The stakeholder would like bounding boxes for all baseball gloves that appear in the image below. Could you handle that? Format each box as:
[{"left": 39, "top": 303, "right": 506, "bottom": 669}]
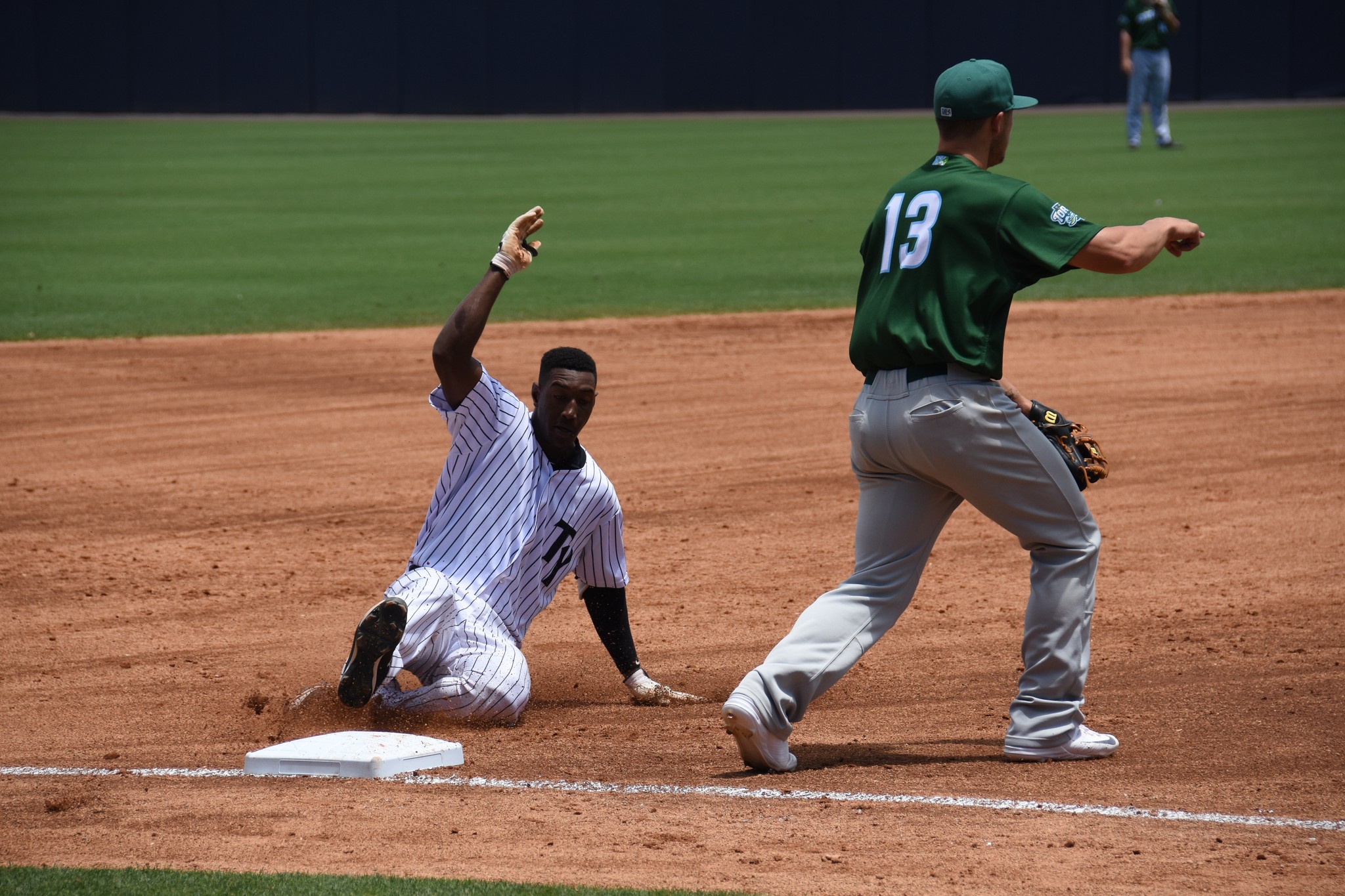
[{"left": 1031, "top": 399, "right": 1106, "bottom": 492}]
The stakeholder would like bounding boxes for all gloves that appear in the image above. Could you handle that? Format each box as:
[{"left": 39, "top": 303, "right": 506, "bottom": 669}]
[
  {"left": 490, "top": 206, "right": 544, "bottom": 280},
  {"left": 622, "top": 668, "right": 706, "bottom": 706}
]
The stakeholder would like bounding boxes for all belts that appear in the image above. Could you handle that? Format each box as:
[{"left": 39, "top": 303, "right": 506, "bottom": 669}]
[{"left": 863, "top": 362, "right": 947, "bottom": 386}]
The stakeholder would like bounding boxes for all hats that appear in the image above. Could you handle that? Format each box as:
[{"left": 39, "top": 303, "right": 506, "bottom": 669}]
[{"left": 933, "top": 59, "right": 1038, "bottom": 121}]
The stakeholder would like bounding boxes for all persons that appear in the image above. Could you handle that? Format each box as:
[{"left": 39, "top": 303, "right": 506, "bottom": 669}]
[
  {"left": 718, "top": 57, "right": 1205, "bottom": 774},
  {"left": 285, "top": 205, "right": 708, "bottom": 725},
  {"left": 1118, "top": 0, "right": 1185, "bottom": 150}
]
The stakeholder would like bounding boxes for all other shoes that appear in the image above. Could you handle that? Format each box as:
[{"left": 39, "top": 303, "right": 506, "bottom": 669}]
[
  {"left": 1129, "top": 143, "right": 1139, "bottom": 150},
  {"left": 1160, "top": 142, "right": 1182, "bottom": 149}
]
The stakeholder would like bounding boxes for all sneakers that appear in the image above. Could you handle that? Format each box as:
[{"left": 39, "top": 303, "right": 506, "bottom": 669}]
[
  {"left": 288, "top": 684, "right": 337, "bottom": 710},
  {"left": 722, "top": 693, "right": 797, "bottom": 773},
  {"left": 338, "top": 597, "right": 408, "bottom": 708},
  {"left": 1004, "top": 723, "right": 1119, "bottom": 762}
]
